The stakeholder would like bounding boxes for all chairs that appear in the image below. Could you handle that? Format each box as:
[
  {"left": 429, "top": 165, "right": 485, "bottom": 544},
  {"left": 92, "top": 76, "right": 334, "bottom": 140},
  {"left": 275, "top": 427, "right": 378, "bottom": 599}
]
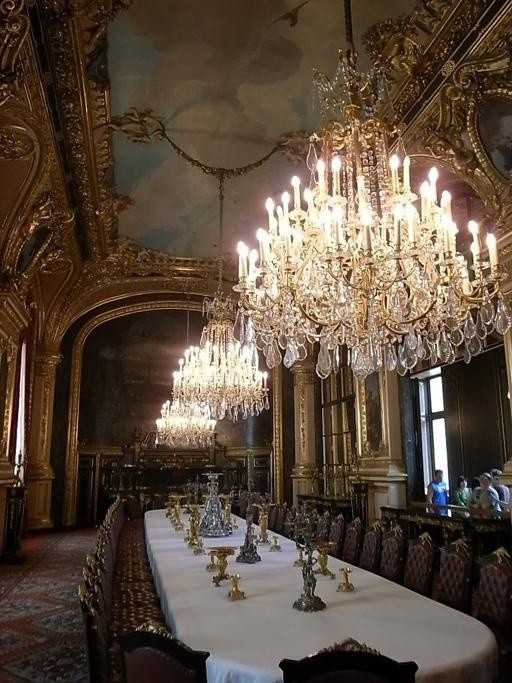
[
  {"left": 231, "top": 499, "right": 383, "bottom": 574},
  {"left": 463, "top": 546, "right": 512, "bottom": 667},
  {"left": 390, "top": 531, "right": 437, "bottom": 598},
  {"left": 116, "top": 619, "right": 210, "bottom": 683},
  {"left": 371, "top": 522, "right": 408, "bottom": 580},
  {"left": 75, "top": 492, "right": 172, "bottom": 683},
  {"left": 279, "top": 638, "right": 418, "bottom": 682},
  {"left": 417, "top": 534, "right": 475, "bottom": 613}
]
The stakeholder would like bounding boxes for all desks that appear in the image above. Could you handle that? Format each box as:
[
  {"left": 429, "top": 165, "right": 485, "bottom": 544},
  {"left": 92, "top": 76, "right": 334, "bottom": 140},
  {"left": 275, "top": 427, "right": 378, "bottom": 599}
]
[
  {"left": 381, "top": 504, "right": 511, "bottom": 586},
  {"left": 144, "top": 508, "right": 500, "bottom": 683}
]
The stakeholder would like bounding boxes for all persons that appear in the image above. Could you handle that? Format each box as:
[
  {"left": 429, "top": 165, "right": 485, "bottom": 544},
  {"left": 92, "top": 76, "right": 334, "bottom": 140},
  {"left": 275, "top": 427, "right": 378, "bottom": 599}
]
[
  {"left": 454, "top": 469, "right": 510, "bottom": 532},
  {"left": 427, "top": 470, "right": 448, "bottom": 517}
]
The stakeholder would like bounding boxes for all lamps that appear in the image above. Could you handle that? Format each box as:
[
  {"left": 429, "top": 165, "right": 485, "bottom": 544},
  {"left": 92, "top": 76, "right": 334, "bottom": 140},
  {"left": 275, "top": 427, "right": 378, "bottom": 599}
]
[{"left": 232, "top": 1, "right": 512, "bottom": 380}]
